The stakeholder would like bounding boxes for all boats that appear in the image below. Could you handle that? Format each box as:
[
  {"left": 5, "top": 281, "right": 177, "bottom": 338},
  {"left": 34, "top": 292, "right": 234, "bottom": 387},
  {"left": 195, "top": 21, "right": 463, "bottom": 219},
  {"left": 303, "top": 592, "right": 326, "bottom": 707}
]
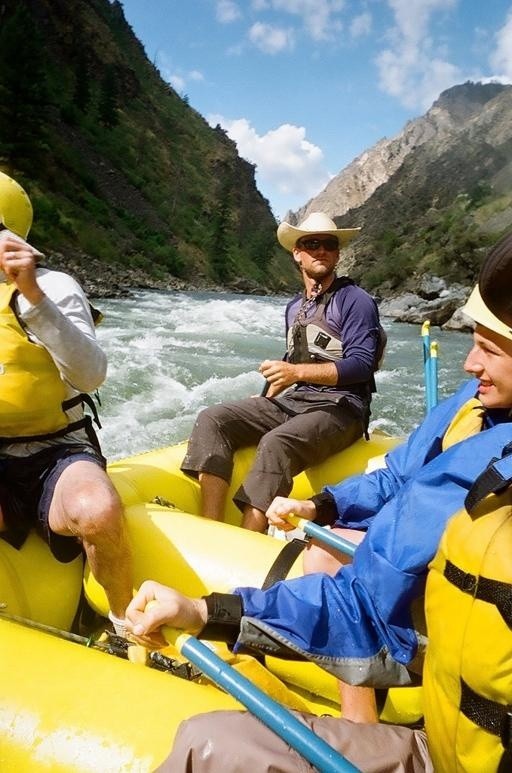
[{"left": 0, "top": 433, "right": 424, "bottom": 772}]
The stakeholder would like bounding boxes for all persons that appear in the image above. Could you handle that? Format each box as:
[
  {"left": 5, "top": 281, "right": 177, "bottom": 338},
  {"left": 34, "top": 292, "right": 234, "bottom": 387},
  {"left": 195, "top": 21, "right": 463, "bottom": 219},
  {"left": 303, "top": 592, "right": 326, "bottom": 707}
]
[
  {"left": 0, "top": 169, "right": 136, "bottom": 638},
  {"left": 123, "top": 420, "right": 512, "bottom": 773},
  {"left": 179, "top": 212, "right": 382, "bottom": 533},
  {"left": 263, "top": 220, "right": 511, "bottom": 725}
]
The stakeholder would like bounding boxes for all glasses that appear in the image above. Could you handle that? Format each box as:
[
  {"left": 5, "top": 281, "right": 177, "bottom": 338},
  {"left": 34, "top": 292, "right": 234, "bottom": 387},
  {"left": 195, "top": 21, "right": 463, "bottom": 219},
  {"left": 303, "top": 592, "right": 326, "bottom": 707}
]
[{"left": 297, "top": 238, "right": 339, "bottom": 251}]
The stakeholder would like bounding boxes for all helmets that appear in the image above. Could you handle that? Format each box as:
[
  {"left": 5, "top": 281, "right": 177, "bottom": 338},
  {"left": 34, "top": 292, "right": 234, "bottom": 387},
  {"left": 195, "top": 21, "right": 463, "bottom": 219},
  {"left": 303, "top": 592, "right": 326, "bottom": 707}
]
[
  {"left": 0, "top": 171, "right": 33, "bottom": 241},
  {"left": 461, "top": 226, "right": 512, "bottom": 339}
]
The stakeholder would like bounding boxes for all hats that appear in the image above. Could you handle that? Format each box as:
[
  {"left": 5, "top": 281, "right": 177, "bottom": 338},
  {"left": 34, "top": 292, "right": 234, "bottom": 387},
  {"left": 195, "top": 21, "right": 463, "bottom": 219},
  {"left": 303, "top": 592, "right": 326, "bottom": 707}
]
[{"left": 277, "top": 211, "right": 362, "bottom": 250}]
